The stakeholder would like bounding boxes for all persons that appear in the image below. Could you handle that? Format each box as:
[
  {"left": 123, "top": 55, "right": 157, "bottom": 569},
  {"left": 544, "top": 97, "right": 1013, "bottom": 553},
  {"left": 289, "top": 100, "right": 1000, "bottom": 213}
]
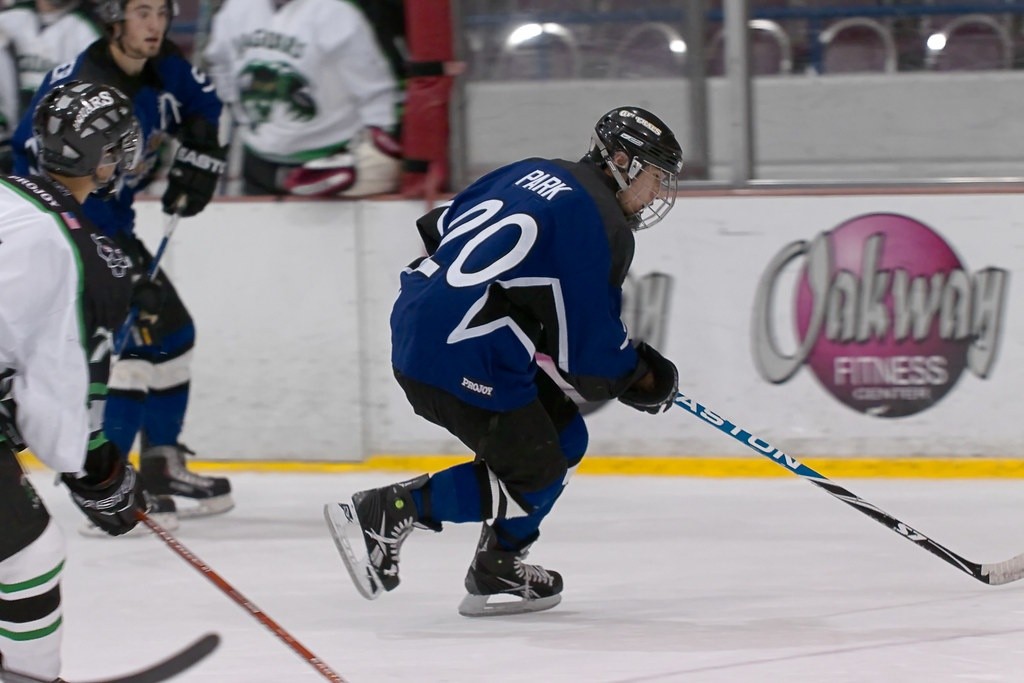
[
  {"left": 324, "top": 105, "right": 684, "bottom": 618},
  {"left": 0, "top": 81, "right": 145, "bottom": 683},
  {"left": 0, "top": 0, "right": 402, "bottom": 197},
  {"left": 12, "top": 0, "right": 234, "bottom": 538}
]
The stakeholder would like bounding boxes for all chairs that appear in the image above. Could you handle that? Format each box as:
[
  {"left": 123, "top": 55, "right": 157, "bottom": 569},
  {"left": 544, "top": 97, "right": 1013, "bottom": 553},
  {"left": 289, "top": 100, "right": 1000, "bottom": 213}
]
[{"left": 491, "top": 14, "right": 1014, "bottom": 79}]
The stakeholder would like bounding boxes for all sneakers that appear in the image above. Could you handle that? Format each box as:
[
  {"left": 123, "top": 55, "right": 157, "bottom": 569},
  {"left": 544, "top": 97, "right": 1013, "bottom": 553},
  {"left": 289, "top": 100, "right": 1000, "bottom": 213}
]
[
  {"left": 78, "top": 490, "right": 179, "bottom": 536},
  {"left": 324, "top": 473, "right": 443, "bottom": 600},
  {"left": 458, "top": 520, "right": 563, "bottom": 616},
  {"left": 139, "top": 443, "right": 233, "bottom": 516}
]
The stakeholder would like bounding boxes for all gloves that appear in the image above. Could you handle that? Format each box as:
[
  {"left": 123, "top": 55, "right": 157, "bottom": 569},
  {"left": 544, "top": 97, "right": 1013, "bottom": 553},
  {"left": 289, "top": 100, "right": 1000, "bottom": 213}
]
[
  {"left": 617, "top": 341, "right": 678, "bottom": 414},
  {"left": 62, "top": 455, "right": 147, "bottom": 536},
  {"left": 161, "top": 138, "right": 230, "bottom": 217}
]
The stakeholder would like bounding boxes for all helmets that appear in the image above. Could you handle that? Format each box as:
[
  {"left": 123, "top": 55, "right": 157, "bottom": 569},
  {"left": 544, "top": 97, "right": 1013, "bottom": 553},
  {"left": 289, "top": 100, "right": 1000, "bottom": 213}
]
[
  {"left": 34, "top": 79, "right": 140, "bottom": 177},
  {"left": 100, "top": 0, "right": 180, "bottom": 24},
  {"left": 591, "top": 106, "right": 683, "bottom": 232}
]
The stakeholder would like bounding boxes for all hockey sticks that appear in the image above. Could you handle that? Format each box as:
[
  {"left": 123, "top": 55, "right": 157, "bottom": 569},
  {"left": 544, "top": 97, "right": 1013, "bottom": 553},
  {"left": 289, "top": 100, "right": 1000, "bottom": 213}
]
[
  {"left": 674, "top": 392, "right": 1024, "bottom": 586},
  {"left": 136, "top": 514, "right": 349, "bottom": 683},
  {"left": 99, "top": 194, "right": 186, "bottom": 379}
]
